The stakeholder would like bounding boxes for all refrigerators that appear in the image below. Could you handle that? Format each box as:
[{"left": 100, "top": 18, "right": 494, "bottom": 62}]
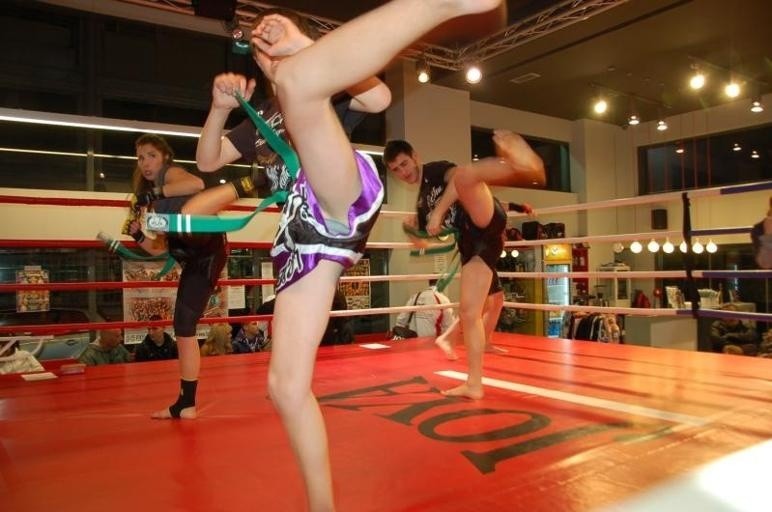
[{"left": 513, "top": 241, "right": 573, "bottom": 337}]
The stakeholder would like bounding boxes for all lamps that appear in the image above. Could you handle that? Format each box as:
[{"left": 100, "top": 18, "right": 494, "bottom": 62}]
[
  {"left": 747, "top": 91, "right": 767, "bottom": 114},
  {"left": 730, "top": 141, "right": 760, "bottom": 160},
  {"left": 610, "top": 108, "right": 722, "bottom": 256},
  {"left": 415, "top": 52, "right": 432, "bottom": 86}
]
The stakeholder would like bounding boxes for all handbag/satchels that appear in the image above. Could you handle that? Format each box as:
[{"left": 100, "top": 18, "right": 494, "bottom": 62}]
[{"left": 391, "top": 325, "right": 417, "bottom": 339}]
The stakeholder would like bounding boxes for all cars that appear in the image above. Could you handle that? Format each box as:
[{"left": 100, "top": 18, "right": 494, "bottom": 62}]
[{"left": 0, "top": 309, "right": 109, "bottom": 363}]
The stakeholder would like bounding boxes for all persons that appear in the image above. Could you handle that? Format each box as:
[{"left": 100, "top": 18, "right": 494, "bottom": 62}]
[
  {"left": 433, "top": 261, "right": 511, "bottom": 362},
  {"left": 195, "top": 1, "right": 502, "bottom": 511},
  {"left": 709, "top": 302, "right": 762, "bottom": 352},
  {"left": 393, "top": 279, "right": 461, "bottom": 337},
  {"left": 321, "top": 289, "right": 356, "bottom": 344},
  {"left": 134, "top": 315, "right": 178, "bottom": 362},
  {"left": 121, "top": 133, "right": 270, "bottom": 421},
  {"left": 750, "top": 196, "right": 772, "bottom": 270},
  {"left": 200, "top": 323, "right": 233, "bottom": 356},
  {"left": 383, "top": 128, "right": 548, "bottom": 402},
  {"left": 232, "top": 312, "right": 270, "bottom": 354},
  {"left": 77, "top": 328, "right": 136, "bottom": 367}
]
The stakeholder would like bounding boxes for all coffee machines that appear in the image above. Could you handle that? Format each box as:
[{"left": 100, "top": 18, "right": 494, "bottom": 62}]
[{"left": 593, "top": 285, "right": 606, "bottom": 306}]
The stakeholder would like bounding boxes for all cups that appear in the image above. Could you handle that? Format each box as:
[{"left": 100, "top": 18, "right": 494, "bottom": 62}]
[
  {"left": 684, "top": 302, "right": 692, "bottom": 309},
  {"left": 604, "top": 301, "right": 609, "bottom": 307}
]
